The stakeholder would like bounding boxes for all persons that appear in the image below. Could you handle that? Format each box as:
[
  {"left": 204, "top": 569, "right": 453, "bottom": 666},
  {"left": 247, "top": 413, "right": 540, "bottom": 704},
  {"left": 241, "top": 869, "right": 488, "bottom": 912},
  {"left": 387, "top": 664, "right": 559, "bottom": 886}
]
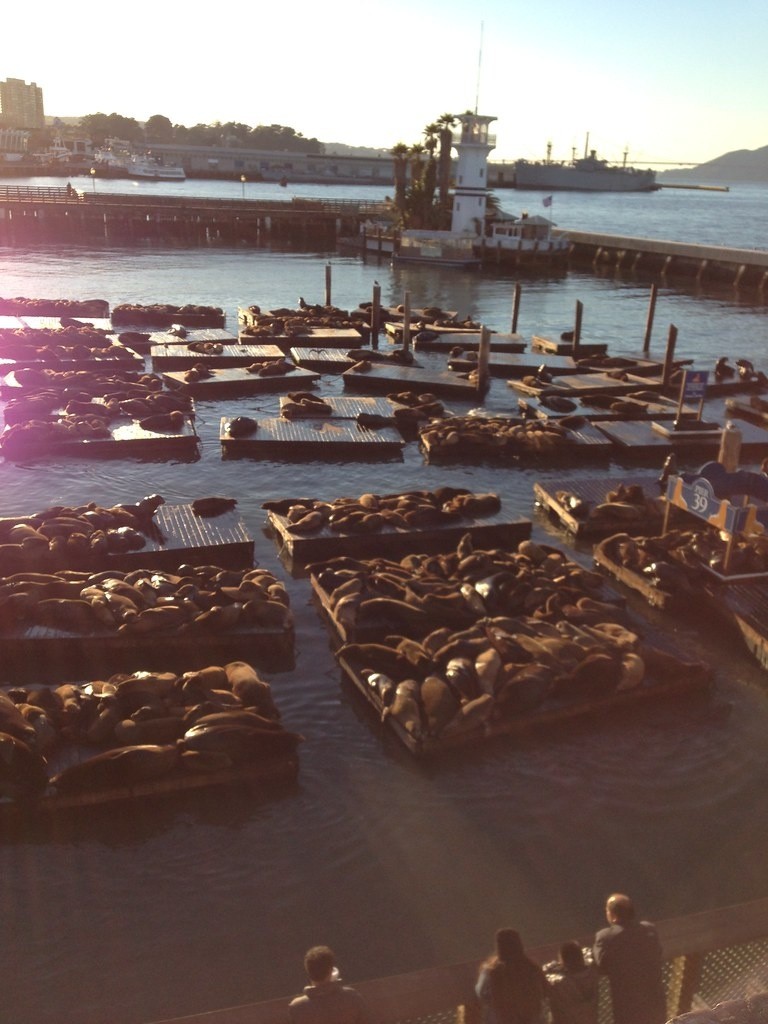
[
  {"left": 475, "top": 927, "right": 547, "bottom": 1024},
  {"left": 540, "top": 940, "right": 601, "bottom": 1024},
  {"left": 289, "top": 943, "right": 366, "bottom": 1024},
  {"left": 593, "top": 893, "right": 668, "bottom": 1024}
]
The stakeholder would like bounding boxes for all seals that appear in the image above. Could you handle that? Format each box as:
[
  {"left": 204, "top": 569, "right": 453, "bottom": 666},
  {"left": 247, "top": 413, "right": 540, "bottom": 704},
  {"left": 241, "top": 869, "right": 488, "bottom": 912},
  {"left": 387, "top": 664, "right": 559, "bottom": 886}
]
[{"left": 0, "top": 294, "right": 767, "bottom": 798}]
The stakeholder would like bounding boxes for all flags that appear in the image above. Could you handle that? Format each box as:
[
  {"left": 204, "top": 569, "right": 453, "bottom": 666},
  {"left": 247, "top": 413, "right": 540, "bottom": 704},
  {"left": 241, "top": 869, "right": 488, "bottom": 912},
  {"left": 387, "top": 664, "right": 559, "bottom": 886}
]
[{"left": 543, "top": 196, "right": 552, "bottom": 206}]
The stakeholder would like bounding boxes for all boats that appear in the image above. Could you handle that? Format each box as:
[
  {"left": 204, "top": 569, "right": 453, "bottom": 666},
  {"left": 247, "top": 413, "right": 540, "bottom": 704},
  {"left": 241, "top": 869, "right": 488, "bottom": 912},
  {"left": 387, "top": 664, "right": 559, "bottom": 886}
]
[
  {"left": 123, "top": 151, "right": 187, "bottom": 182},
  {"left": 515, "top": 132, "right": 656, "bottom": 193}
]
